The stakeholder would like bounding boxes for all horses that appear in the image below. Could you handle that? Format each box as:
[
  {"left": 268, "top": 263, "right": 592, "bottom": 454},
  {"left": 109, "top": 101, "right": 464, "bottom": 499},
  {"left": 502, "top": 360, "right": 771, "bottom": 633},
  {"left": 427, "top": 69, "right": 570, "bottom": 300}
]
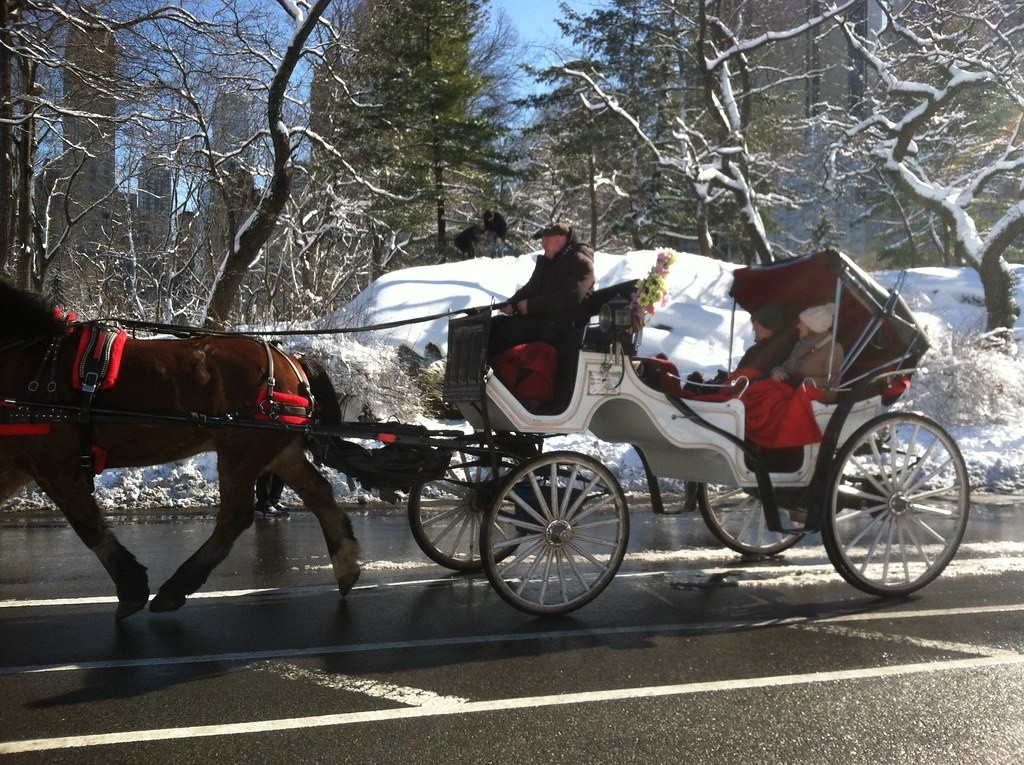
[{"left": 1, "top": 272, "right": 369, "bottom": 620}]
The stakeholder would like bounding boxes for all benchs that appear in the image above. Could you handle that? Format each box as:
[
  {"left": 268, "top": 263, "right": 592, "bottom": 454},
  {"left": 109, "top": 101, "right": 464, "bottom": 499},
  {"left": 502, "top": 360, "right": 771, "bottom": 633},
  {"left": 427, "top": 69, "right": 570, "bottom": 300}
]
[
  {"left": 554, "top": 279, "right": 642, "bottom": 392},
  {"left": 768, "top": 359, "right": 895, "bottom": 471}
]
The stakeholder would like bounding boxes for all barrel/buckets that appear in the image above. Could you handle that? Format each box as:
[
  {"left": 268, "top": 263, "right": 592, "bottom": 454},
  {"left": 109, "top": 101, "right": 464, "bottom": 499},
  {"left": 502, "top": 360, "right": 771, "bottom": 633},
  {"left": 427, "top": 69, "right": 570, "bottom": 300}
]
[{"left": 515, "top": 462, "right": 588, "bottom": 533}]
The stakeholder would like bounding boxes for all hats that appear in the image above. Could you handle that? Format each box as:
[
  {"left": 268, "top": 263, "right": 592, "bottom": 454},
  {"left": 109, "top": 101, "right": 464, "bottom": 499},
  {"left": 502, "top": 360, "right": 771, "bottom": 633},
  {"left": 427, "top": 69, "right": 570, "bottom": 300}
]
[
  {"left": 799, "top": 302, "right": 835, "bottom": 333},
  {"left": 532, "top": 222, "right": 570, "bottom": 239},
  {"left": 751, "top": 304, "right": 783, "bottom": 331}
]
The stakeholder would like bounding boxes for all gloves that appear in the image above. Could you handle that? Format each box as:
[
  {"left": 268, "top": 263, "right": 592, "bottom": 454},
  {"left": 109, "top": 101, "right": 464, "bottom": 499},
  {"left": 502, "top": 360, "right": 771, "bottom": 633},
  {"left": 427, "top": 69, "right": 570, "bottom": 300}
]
[{"left": 772, "top": 370, "right": 788, "bottom": 381}]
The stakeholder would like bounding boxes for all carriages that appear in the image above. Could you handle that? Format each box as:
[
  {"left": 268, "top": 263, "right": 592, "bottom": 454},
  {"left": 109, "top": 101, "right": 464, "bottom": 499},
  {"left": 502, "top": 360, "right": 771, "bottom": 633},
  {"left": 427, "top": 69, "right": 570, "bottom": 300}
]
[{"left": 0, "top": 242, "right": 974, "bottom": 624}]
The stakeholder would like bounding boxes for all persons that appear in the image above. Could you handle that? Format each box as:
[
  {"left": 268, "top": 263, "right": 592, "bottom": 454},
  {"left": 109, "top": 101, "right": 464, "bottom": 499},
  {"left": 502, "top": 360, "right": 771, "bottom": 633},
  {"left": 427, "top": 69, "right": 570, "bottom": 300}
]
[
  {"left": 771, "top": 303, "right": 845, "bottom": 402},
  {"left": 488, "top": 224, "right": 597, "bottom": 366},
  {"left": 454, "top": 224, "right": 484, "bottom": 259},
  {"left": 255, "top": 469, "right": 290, "bottom": 517},
  {"left": 483, "top": 209, "right": 507, "bottom": 258},
  {"left": 684, "top": 299, "right": 800, "bottom": 395}
]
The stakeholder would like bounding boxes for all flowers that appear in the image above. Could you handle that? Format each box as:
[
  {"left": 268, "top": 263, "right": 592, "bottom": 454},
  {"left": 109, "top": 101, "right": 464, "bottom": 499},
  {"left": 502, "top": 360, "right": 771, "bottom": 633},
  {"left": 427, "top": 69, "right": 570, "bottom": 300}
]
[{"left": 628, "top": 250, "right": 676, "bottom": 331}]
